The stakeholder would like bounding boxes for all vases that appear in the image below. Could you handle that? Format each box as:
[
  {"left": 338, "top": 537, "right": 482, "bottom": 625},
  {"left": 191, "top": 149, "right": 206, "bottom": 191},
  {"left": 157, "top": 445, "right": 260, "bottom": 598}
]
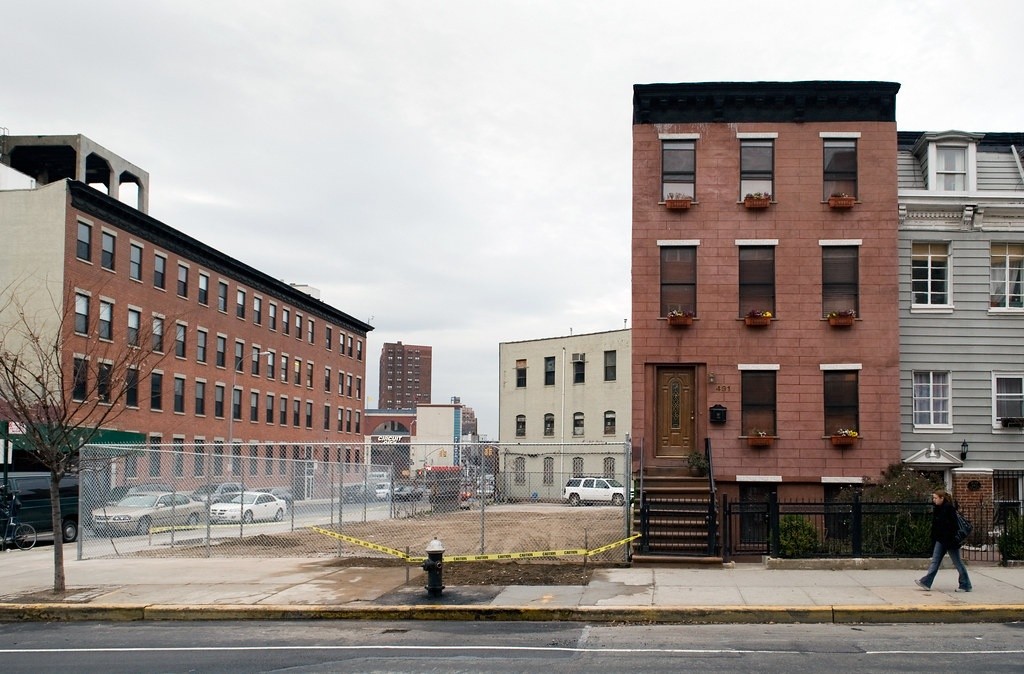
[
  {"left": 829, "top": 317, "right": 854, "bottom": 326},
  {"left": 667, "top": 317, "right": 693, "bottom": 325},
  {"left": 744, "top": 199, "right": 771, "bottom": 207},
  {"left": 827, "top": 197, "right": 855, "bottom": 207},
  {"left": 830, "top": 436, "right": 857, "bottom": 445},
  {"left": 744, "top": 317, "right": 771, "bottom": 325},
  {"left": 747, "top": 436, "right": 773, "bottom": 446}
]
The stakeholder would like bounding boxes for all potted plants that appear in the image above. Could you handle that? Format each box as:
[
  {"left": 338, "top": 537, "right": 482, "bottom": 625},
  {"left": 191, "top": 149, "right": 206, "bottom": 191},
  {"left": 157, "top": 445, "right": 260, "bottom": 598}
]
[
  {"left": 682, "top": 452, "right": 710, "bottom": 476},
  {"left": 666, "top": 193, "right": 693, "bottom": 209}
]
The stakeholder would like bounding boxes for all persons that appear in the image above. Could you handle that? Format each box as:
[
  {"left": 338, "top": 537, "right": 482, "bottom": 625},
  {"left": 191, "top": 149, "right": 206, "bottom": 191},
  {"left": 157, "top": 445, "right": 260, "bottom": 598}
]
[{"left": 915, "top": 489, "right": 973, "bottom": 593}]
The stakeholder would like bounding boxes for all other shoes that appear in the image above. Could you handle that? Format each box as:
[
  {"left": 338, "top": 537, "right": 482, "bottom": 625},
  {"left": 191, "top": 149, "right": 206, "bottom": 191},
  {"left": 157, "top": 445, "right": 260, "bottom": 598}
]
[
  {"left": 914, "top": 579, "right": 930, "bottom": 591},
  {"left": 955, "top": 588, "right": 972, "bottom": 592}
]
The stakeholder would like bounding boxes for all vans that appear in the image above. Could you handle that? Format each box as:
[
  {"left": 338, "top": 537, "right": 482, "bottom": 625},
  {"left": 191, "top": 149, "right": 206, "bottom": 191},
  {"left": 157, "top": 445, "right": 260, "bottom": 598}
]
[{"left": 0, "top": 471, "right": 79, "bottom": 543}]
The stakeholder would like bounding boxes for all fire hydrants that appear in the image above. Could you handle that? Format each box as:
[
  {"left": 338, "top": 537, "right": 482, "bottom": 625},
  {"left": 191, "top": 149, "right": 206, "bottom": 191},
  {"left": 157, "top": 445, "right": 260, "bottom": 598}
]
[{"left": 420, "top": 536, "right": 446, "bottom": 601}]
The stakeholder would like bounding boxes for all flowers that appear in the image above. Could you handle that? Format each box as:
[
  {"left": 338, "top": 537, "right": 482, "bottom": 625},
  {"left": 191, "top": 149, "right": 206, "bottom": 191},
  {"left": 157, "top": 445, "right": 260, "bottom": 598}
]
[
  {"left": 666, "top": 309, "right": 696, "bottom": 318},
  {"left": 832, "top": 428, "right": 858, "bottom": 437},
  {"left": 827, "top": 309, "right": 857, "bottom": 318},
  {"left": 830, "top": 192, "right": 849, "bottom": 198},
  {"left": 749, "top": 427, "right": 766, "bottom": 437},
  {"left": 746, "top": 308, "right": 772, "bottom": 316},
  {"left": 744, "top": 192, "right": 772, "bottom": 200}
]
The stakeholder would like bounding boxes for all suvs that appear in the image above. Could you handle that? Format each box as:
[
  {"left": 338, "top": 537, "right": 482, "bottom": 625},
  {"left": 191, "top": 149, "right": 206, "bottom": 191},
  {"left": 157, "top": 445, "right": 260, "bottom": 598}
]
[{"left": 562, "top": 475, "right": 634, "bottom": 507}]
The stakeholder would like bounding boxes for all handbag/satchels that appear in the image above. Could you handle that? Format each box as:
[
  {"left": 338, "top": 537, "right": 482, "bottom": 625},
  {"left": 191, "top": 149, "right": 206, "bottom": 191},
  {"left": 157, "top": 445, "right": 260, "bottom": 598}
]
[{"left": 954, "top": 513, "right": 973, "bottom": 544}]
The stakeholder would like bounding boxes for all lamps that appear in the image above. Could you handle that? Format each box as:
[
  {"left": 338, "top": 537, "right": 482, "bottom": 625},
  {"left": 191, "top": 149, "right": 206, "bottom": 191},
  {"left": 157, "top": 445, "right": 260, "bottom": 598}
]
[{"left": 959, "top": 439, "right": 968, "bottom": 461}]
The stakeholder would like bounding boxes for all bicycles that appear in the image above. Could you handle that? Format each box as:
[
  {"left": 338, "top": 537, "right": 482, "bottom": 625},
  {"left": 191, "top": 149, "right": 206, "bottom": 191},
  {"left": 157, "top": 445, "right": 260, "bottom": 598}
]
[{"left": 0, "top": 484, "right": 38, "bottom": 551}]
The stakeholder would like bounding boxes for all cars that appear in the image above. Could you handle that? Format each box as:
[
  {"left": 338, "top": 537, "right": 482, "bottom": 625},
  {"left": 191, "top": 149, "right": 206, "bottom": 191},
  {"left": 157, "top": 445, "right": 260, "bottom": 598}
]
[
  {"left": 190, "top": 481, "right": 293, "bottom": 510},
  {"left": 339, "top": 481, "right": 424, "bottom": 504},
  {"left": 208, "top": 491, "right": 287, "bottom": 524},
  {"left": 461, "top": 474, "right": 494, "bottom": 501},
  {"left": 91, "top": 490, "right": 206, "bottom": 538}
]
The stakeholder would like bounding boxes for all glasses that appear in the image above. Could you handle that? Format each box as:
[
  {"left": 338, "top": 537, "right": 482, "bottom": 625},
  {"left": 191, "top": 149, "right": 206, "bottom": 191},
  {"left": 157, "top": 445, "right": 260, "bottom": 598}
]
[{"left": 933, "top": 496, "right": 940, "bottom": 499}]
